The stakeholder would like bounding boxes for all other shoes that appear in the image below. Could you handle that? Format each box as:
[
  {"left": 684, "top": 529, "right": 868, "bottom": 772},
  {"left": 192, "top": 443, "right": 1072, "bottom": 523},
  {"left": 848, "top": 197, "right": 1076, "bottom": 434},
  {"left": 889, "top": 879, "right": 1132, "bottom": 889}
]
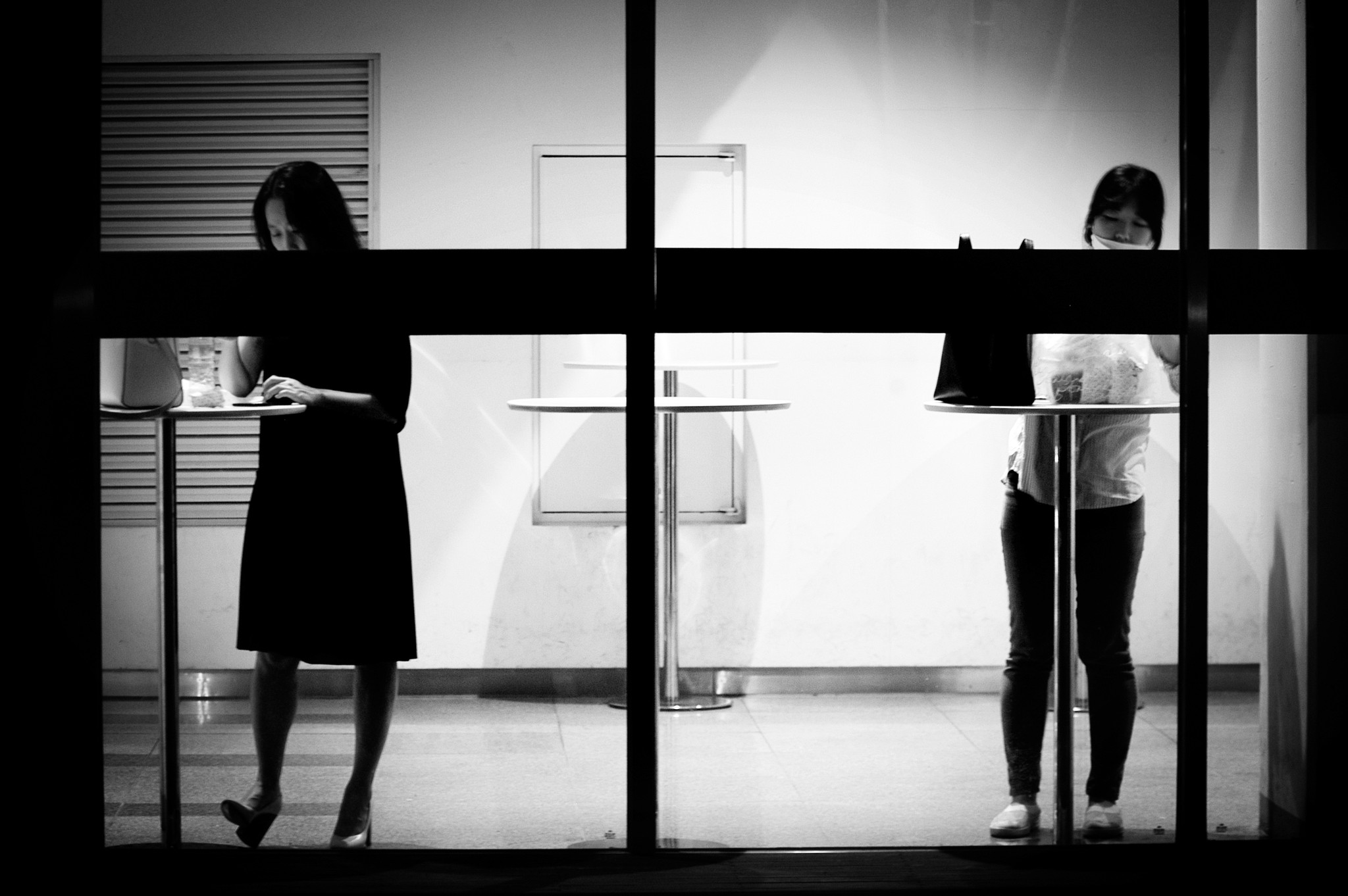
[
  {"left": 1083, "top": 800, "right": 1124, "bottom": 838},
  {"left": 989, "top": 800, "right": 1041, "bottom": 838}
]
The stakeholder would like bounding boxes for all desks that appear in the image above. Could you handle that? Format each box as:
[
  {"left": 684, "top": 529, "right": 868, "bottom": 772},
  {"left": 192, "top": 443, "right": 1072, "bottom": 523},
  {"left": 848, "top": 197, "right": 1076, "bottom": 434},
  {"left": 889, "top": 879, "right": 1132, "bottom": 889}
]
[
  {"left": 510, "top": 401, "right": 791, "bottom": 849},
  {"left": 99, "top": 402, "right": 307, "bottom": 843},
  {"left": 507, "top": 364, "right": 792, "bottom": 712},
  {"left": 925, "top": 402, "right": 1180, "bottom": 845}
]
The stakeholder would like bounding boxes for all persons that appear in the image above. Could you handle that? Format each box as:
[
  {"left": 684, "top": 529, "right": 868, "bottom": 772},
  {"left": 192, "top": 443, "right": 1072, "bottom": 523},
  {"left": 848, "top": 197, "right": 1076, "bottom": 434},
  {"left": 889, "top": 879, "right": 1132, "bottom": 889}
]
[
  {"left": 217, "top": 161, "right": 418, "bottom": 852},
  {"left": 987, "top": 162, "right": 1178, "bottom": 839}
]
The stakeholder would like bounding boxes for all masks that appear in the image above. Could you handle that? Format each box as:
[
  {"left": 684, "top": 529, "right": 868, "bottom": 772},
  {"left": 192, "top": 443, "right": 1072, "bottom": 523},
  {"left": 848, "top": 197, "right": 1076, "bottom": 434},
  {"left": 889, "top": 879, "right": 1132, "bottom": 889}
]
[{"left": 1089, "top": 234, "right": 1155, "bottom": 250}]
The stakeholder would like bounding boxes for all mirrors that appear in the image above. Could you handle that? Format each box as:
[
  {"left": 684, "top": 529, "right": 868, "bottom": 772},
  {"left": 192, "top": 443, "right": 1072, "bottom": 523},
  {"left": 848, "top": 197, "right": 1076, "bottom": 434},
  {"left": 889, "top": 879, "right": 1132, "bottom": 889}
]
[{"left": 532, "top": 144, "right": 746, "bottom": 525}]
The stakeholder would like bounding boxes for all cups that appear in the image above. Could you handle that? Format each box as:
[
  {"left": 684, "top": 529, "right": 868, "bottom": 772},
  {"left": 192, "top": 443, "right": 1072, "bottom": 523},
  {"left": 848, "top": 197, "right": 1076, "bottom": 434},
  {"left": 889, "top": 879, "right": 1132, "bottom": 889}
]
[{"left": 1048, "top": 345, "right": 1083, "bottom": 405}]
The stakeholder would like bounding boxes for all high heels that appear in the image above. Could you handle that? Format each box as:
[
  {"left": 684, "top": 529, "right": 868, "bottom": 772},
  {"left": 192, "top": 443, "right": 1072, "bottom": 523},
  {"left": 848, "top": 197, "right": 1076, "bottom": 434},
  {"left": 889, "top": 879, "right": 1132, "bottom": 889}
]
[
  {"left": 220, "top": 794, "right": 284, "bottom": 847},
  {"left": 329, "top": 799, "right": 373, "bottom": 849}
]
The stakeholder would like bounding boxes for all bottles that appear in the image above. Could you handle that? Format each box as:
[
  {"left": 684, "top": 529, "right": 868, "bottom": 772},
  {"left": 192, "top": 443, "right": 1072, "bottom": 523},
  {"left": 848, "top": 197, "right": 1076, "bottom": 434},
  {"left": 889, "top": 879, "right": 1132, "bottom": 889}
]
[{"left": 188, "top": 336, "right": 214, "bottom": 397}]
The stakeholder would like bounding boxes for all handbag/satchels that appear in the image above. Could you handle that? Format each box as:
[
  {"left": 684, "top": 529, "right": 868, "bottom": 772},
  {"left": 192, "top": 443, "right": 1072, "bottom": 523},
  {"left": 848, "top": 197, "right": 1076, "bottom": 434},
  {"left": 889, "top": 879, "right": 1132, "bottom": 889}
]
[
  {"left": 99, "top": 336, "right": 185, "bottom": 419},
  {"left": 934, "top": 235, "right": 1037, "bottom": 407}
]
